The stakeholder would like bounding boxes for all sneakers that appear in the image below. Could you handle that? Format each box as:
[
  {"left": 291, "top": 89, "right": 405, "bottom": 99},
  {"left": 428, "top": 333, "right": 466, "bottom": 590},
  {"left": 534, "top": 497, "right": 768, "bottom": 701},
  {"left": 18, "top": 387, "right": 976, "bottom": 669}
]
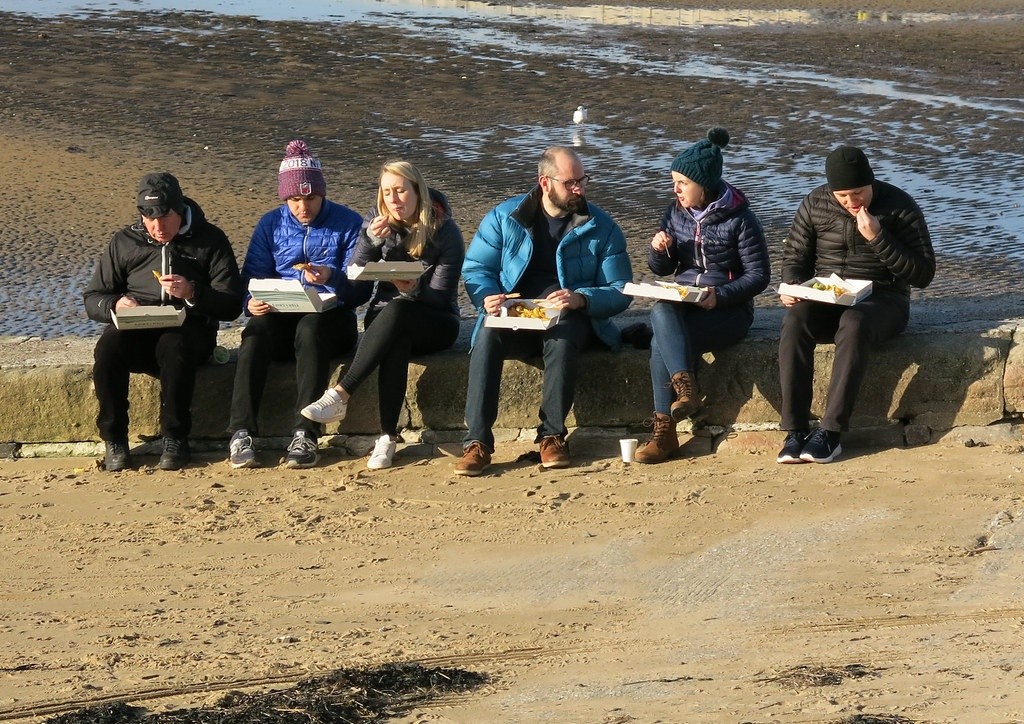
[
  {"left": 286, "top": 429, "right": 322, "bottom": 468},
  {"left": 798, "top": 426, "right": 842, "bottom": 464},
  {"left": 105, "top": 436, "right": 132, "bottom": 471},
  {"left": 540, "top": 433, "right": 570, "bottom": 468},
  {"left": 368, "top": 435, "right": 396, "bottom": 469},
  {"left": 301, "top": 388, "right": 348, "bottom": 424},
  {"left": 455, "top": 441, "right": 491, "bottom": 475},
  {"left": 160, "top": 436, "right": 187, "bottom": 471},
  {"left": 777, "top": 429, "right": 811, "bottom": 463},
  {"left": 229, "top": 428, "right": 256, "bottom": 468}
]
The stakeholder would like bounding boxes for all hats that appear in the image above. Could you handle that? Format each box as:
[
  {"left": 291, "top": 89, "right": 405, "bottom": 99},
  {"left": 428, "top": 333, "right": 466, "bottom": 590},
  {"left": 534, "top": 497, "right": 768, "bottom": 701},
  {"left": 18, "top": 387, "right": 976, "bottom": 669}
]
[
  {"left": 137, "top": 171, "right": 185, "bottom": 219},
  {"left": 670, "top": 127, "right": 730, "bottom": 192},
  {"left": 825, "top": 147, "right": 875, "bottom": 191},
  {"left": 278, "top": 140, "right": 326, "bottom": 201}
]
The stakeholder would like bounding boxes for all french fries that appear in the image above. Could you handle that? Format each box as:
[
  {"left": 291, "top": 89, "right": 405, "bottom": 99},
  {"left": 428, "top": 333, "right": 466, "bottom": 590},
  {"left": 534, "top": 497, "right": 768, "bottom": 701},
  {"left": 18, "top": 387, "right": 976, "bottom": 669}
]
[
  {"left": 677, "top": 287, "right": 688, "bottom": 299},
  {"left": 825, "top": 285, "right": 842, "bottom": 299},
  {"left": 519, "top": 307, "right": 547, "bottom": 319},
  {"left": 152, "top": 270, "right": 161, "bottom": 281}
]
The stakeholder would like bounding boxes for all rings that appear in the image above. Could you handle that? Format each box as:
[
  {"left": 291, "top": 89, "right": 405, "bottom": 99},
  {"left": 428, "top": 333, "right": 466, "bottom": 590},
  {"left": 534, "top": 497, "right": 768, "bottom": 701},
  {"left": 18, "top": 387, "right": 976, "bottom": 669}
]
[
  {"left": 173, "top": 287, "right": 176, "bottom": 292},
  {"left": 708, "top": 303, "right": 712, "bottom": 307},
  {"left": 377, "top": 235, "right": 380, "bottom": 239}
]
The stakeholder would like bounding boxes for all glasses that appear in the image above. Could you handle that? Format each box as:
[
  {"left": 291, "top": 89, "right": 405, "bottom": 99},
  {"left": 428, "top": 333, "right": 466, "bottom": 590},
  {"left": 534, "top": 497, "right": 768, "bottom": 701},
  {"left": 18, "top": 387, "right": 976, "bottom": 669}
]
[{"left": 543, "top": 176, "right": 589, "bottom": 190}]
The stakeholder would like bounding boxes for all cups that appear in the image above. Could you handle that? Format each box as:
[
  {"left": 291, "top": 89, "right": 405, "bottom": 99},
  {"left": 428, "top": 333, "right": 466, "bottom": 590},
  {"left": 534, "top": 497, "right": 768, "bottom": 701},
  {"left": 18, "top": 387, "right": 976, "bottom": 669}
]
[{"left": 619, "top": 438, "right": 638, "bottom": 463}]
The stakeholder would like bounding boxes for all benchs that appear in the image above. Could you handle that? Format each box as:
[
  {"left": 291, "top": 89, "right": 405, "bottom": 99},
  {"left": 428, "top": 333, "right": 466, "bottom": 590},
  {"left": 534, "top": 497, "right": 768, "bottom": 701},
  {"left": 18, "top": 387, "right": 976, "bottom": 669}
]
[{"left": 0, "top": 316, "right": 1023, "bottom": 445}]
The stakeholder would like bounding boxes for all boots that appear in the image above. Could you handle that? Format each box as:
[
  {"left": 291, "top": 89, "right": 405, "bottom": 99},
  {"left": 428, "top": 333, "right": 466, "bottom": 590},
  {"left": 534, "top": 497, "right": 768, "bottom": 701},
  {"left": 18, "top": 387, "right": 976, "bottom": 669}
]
[
  {"left": 634, "top": 411, "right": 679, "bottom": 463},
  {"left": 663, "top": 370, "right": 704, "bottom": 422}
]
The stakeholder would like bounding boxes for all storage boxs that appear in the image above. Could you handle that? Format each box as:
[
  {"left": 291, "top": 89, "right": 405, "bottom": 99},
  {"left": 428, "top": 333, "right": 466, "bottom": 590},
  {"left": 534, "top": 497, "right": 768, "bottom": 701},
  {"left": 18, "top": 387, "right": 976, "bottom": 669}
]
[
  {"left": 483, "top": 298, "right": 569, "bottom": 331},
  {"left": 778, "top": 277, "right": 873, "bottom": 306},
  {"left": 347, "top": 261, "right": 433, "bottom": 280},
  {"left": 622, "top": 280, "right": 710, "bottom": 303},
  {"left": 109, "top": 306, "right": 186, "bottom": 330},
  {"left": 248, "top": 278, "right": 338, "bottom": 313}
]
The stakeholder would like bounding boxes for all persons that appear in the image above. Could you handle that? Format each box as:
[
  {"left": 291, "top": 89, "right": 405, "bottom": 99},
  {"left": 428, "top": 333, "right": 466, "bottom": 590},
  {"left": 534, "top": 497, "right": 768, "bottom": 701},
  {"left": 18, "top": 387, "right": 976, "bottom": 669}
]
[
  {"left": 777, "top": 145, "right": 936, "bottom": 463},
  {"left": 635, "top": 127, "right": 771, "bottom": 463},
  {"left": 83, "top": 173, "right": 244, "bottom": 471},
  {"left": 454, "top": 145, "right": 633, "bottom": 476},
  {"left": 299, "top": 161, "right": 465, "bottom": 470},
  {"left": 228, "top": 140, "right": 374, "bottom": 470}
]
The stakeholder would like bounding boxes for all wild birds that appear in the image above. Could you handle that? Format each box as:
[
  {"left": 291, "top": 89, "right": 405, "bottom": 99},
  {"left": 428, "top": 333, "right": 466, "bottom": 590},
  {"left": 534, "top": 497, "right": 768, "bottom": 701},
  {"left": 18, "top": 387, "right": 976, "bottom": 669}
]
[
  {"left": 572, "top": 131, "right": 587, "bottom": 147},
  {"left": 572, "top": 105, "right": 588, "bottom": 125}
]
